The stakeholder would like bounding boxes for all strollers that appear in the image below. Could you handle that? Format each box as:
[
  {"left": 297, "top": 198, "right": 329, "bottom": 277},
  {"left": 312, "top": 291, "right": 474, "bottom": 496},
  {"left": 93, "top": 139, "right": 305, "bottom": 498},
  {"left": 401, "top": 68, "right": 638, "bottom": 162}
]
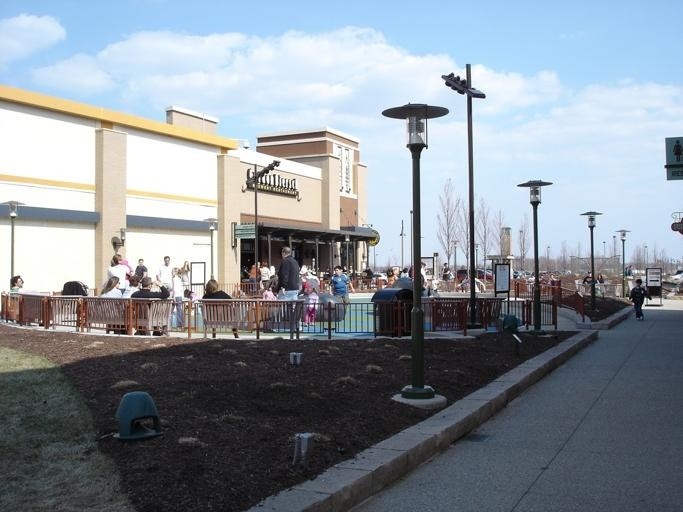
[{"left": 61, "top": 281, "right": 87, "bottom": 296}]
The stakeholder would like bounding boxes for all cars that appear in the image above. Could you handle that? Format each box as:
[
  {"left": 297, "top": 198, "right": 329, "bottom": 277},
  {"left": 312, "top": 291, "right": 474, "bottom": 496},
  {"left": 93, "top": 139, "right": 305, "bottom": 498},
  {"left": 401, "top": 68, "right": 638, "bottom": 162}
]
[{"left": 456, "top": 269, "right": 547, "bottom": 281}]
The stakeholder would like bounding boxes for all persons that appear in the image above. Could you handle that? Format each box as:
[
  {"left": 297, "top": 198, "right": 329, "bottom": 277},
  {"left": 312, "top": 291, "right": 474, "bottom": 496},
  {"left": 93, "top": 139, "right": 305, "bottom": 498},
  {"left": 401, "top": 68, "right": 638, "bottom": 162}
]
[
  {"left": 97, "top": 276, "right": 126, "bottom": 334},
  {"left": 108, "top": 253, "right": 132, "bottom": 294},
  {"left": 441, "top": 262, "right": 452, "bottom": 292},
  {"left": 348, "top": 265, "right": 354, "bottom": 274},
  {"left": 133, "top": 258, "right": 148, "bottom": 287},
  {"left": 245, "top": 258, "right": 331, "bottom": 331},
  {"left": 266, "top": 245, "right": 302, "bottom": 332},
  {"left": 627, "top": 279, "right": 652, "bottom": 321},
  {"left": 362, "top": 265, "right": 372, "bottom": 285},
  {"left": 127, "top": 275, "right": 170, "bottom": 335},
  {"left": 200, "top": 279, "right": 240, "bottom": 338},
  {"left": 379, "top": 259, "right": 440, "bottom": 298},
  {"left": 627, "top": 265, "right": 634, "bottom": 277},
  {"left": 179, "top": 260, "right": 191, "bottom": 288},
  {"left": 623, "top": 267, "right": 628, "bottom": 276},
  {"left": 8, "top": 275, "right": 24, "bottom": 293},
  {"left": 167, "top": 267, "right": 183, "bottom": 332},
  {"left": 121, "top": 275, "right": 140, "bottom": 319},
  {"left": 580, "top": 271, "right": 593, "bottom": 296},
  {"left": 526, "top": 271, "right": 557, "bottom": 295},
  {"left": 341, "top": 265, "right": 348, "bottom": 275},
  {"left": 595, "top": 273, "right": 604, "bottom": 284},
  {"left": 455, "top": 268, "right": 487, "bottom": 293},
  {"left": 331, "top": 265, "right": 356, "bottom": 318},
  {"left": 153, "top": 255, "right": 173, "bottom": 291}
]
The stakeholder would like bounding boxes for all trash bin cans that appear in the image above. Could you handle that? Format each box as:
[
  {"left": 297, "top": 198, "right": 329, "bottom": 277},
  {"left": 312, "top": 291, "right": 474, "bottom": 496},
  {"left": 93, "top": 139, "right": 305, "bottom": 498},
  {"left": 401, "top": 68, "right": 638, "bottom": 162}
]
[
  {"left": 371, "top": 288, "right": 413, "bottom": 336},
  {"left": 61, "top": 281, "right": 89, "bottom": 313}
]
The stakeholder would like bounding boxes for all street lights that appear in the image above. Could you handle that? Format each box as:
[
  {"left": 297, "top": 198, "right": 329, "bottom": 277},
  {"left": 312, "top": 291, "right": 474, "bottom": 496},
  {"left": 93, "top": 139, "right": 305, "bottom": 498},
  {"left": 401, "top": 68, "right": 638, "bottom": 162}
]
[
  {"left": 616, "top": 230, "right": 631, "bottom": 298},
  {"left": 518, "top": 180, "right": 553, "bottom": 336},
  {"left": 247, "top": 161, "right": 281, "bottom": 283},
  {"left": 205, "top": 218, "right": 221, "bottom": 280},
  {"left": 2, "top": 201, "right": 24, "bottom": 290},
  {"left": 382, "top": 102, "right": 449, "bottom": 399},
  {"left": 580, "top": 211, "right": 601, "bottom": 322},
  {"left": 441, "top": 64, "right": 482, "bottom": 328}
]
